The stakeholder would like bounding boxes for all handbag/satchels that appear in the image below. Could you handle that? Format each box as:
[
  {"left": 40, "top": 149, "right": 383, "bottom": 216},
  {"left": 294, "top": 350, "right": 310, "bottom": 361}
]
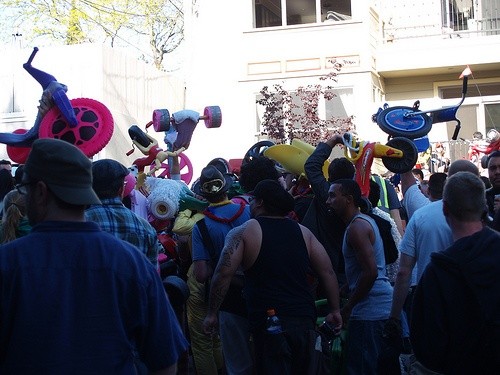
[{"left": 370, "top": 213, "right": 398, "bottom": 265}]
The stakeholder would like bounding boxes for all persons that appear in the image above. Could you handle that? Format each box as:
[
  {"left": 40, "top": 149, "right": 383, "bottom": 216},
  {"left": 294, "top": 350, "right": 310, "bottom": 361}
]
[
  {"left": 0, "top": 160, "right": 33, "bottom": 243},
  {"left": 172, "top": 129, "right": 500, "bottom": 375},
  {"left": 84, "top": 160, "right": 160, "bottom": 272},
  {"left": 0, "top": 138, "right": 190, "bottom": 375},
  {"left": 202, "top": 179, "right": 342, "bottom": 375}
]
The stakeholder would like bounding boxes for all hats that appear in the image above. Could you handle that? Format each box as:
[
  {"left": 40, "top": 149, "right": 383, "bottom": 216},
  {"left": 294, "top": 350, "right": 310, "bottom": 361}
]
[
  {"left": 22, "top": 138, "right": 103, "bottom": 206},
  {"left": 193, "top": 165, "right": 233, "bottom": 199},
  {"left": 92, "top": 158, "right": 130, "bottom": 190},
  {"left": 247, "top": 178, "right": 279, "bottom": 201}
]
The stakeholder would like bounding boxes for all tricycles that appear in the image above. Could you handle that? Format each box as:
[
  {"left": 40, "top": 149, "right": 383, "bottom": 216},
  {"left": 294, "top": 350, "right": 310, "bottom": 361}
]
[
  {"left": 125, "top": 105, "right": 223, "bottom": 186},
  {"left": 371, "top": 75, "right": 468, "bottom": 174},
  {"left": 1, "top": 47, "right": 115, "bottom": 158}
]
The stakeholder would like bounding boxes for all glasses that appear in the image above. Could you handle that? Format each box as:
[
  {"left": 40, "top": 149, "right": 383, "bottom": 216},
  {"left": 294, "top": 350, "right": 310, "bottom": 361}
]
[{"left": 15, "top": 180, "right": 30, "bottom": 196}]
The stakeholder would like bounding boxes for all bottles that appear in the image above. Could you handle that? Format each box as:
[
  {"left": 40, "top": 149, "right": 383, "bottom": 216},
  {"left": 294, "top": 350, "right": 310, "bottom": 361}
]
[{"left": 265, "top": 308, "right": 283, "bottom": 335}]
